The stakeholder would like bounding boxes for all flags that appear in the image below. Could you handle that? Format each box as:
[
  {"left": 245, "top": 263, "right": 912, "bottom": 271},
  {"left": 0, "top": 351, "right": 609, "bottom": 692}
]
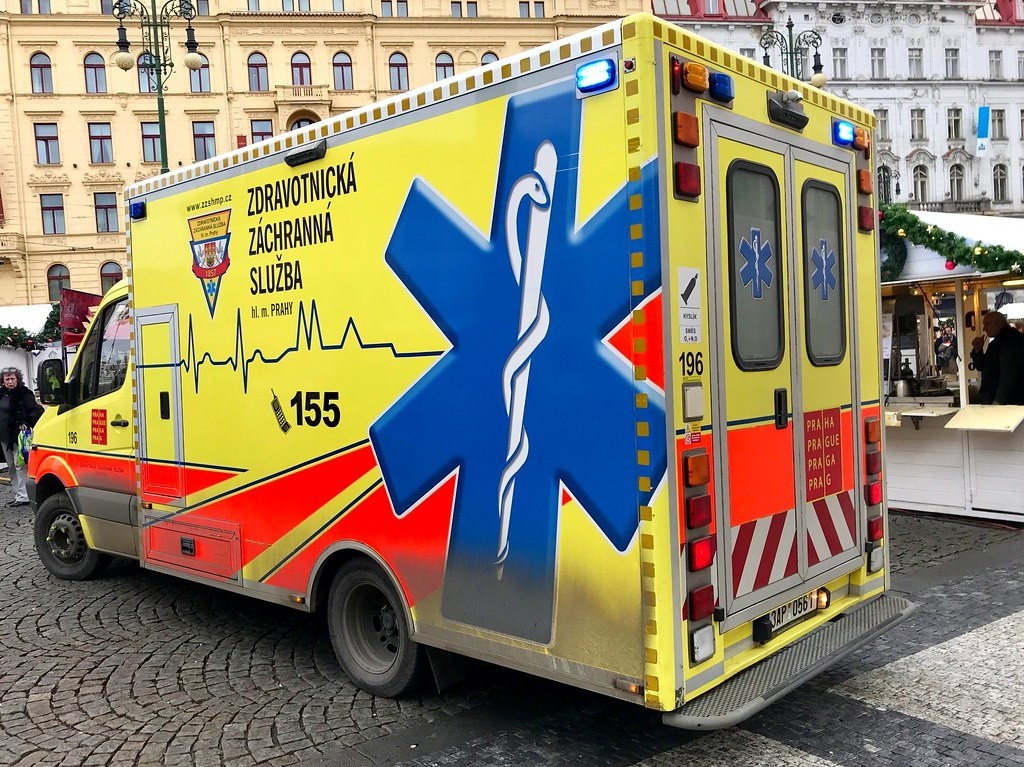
[{"left": 59, "top": 286, "right": 105, "bottom": 348}]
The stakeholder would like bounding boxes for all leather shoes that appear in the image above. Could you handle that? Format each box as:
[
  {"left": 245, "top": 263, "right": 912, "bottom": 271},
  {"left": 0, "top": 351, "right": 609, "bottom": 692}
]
[{"left": 7, "top": 499, "right": 31, "bottom": 507}]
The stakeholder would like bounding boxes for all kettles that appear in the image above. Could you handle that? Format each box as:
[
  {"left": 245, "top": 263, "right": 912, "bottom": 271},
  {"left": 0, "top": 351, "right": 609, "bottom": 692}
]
[{"left": 894, "top": 378, "right": 920, "bottom": 397}]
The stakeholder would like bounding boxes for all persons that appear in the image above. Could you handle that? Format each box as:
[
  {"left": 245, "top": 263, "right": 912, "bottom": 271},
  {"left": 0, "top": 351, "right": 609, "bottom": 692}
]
[
  {"left": 1015, "top": 322, "right": 1024, "bottom": 332},
  {"left": 42, "top": 368, "right": 62, "bottom": 403},
  {"left": 0, "top": 367, "right": 44, "bottom": 508},
  {"left": 934, "top": 321, "right": 958, "bottom": 375},
  {"left": 971, "top": 310, "right": 1024, "bottom": 406}
]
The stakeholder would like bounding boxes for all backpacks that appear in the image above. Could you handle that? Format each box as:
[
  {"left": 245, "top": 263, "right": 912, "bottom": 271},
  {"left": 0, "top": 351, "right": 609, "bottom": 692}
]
[{"left": 938, "top": 341, "right": 954, "bottom": 368}]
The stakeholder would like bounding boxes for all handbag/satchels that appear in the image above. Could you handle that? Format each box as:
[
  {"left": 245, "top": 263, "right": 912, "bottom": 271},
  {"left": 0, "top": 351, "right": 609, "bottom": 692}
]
[{"left": 17, "top": 430, "right": 32, "bottom": 467}]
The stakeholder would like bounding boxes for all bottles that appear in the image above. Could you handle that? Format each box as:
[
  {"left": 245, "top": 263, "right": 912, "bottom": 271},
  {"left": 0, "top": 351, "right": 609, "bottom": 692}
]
[{"left": 901, "top": 358, "right": 913, "bottom": 378}]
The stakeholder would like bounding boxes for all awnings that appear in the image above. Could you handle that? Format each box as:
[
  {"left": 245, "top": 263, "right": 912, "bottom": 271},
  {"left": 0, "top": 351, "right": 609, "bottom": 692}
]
[{"left": 993, "top": 302, "right": 1024, "bottom": 320}]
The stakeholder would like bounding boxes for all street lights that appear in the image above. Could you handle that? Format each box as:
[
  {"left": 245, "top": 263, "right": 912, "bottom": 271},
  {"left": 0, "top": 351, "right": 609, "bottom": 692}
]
[{"left": 113, "top": 1, "right": 203, "bottom": 174}]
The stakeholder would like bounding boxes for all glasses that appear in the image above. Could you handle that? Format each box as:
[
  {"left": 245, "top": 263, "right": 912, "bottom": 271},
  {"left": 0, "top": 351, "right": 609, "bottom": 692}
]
[{"left": 2, "top": 368, "right": 17, "bottom": 373}]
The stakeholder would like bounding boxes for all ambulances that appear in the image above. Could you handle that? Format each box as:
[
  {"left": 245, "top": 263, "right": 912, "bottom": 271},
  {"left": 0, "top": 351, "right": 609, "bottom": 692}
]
[{"left": 25, "top": 12, "right": 918, "bottom": 731}]
